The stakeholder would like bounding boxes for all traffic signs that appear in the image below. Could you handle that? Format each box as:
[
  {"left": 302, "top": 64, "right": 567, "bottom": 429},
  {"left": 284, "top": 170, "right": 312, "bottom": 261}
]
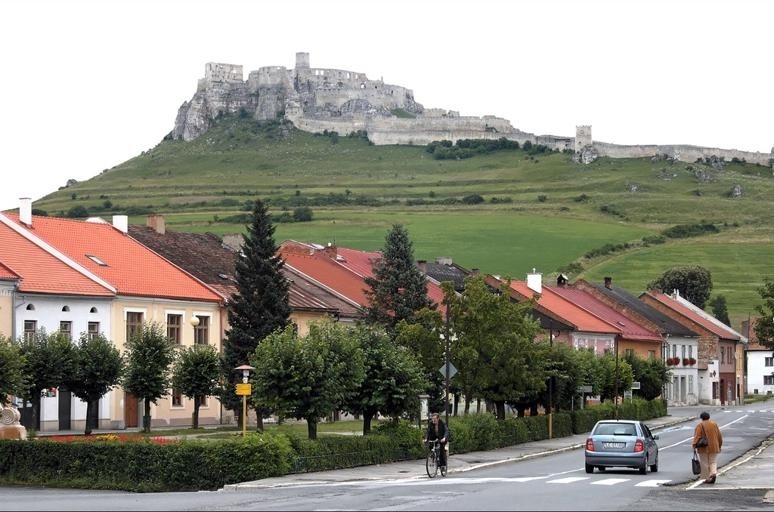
[
  {"left": 234, "top": 384, "right": 251, "bottom": 390},
  {"left": 234, "top": 389, "right": 251, "bottom": 396}
]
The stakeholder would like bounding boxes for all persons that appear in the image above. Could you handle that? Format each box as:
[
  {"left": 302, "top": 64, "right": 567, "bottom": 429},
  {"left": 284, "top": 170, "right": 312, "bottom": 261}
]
[
  {"left": 691, "top": 412, "right": 723, "bottom": 483},
  {"left": 423, "top": 412, "right": 449, "bottom": 472}
]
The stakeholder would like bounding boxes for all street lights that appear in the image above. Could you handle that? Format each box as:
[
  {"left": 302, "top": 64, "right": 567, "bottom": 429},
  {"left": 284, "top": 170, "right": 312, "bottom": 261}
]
[{"left": 189, "top": 317, "right": 200, "bottom": 427}]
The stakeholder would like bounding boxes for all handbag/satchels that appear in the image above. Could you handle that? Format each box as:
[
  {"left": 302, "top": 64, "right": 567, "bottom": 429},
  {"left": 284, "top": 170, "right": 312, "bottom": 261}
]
[
  {"left": 691, "top": 459, "right": 701, "bottom": 474},
  {"left": 695, "top": 438, "right": 708, "bottom": 448}
]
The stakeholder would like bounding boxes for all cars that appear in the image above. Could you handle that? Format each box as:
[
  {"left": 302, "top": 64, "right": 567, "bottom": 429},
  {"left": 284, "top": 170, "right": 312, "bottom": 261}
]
[{"left": 585, "top": 419, "right": 660, "bottom": 474}]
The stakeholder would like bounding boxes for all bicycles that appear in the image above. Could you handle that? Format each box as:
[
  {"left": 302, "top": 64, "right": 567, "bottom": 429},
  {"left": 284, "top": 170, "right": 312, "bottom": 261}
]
[{"left": 424, "top": 439, "right": 447, "bottom": 478}]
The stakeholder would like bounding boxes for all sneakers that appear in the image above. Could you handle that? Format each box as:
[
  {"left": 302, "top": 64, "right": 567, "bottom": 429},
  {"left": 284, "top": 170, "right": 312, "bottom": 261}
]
[
  {"left": 704, "top": 475, "right": 716, "bottom": 484},
  {"left": 441, "top": 466, "right": 446, "bottom": 472}
]
